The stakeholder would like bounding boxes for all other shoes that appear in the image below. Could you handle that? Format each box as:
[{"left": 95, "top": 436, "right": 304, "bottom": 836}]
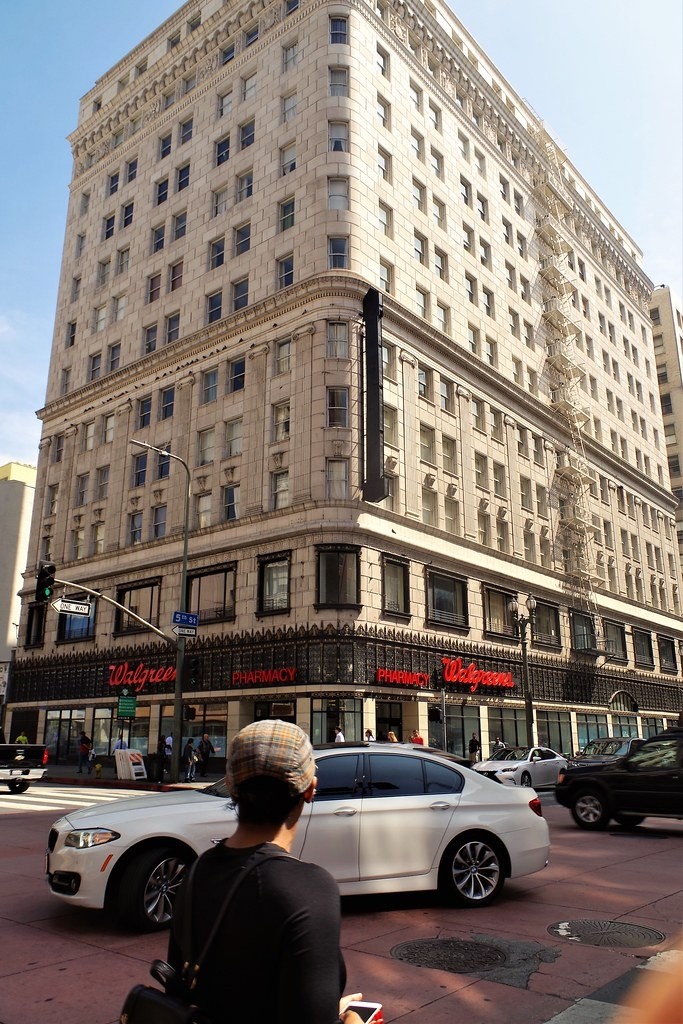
[
  {"left": 191, "top": 779, "right": 197, "bottom": 782},
  {"left": 200, "top": 774, "right": 208, "bottom": 777},
  {"left": 184, "top": 779, "right": 190, "bottom": 783},
  {"left": 77, "top": 771, "right": 82, "bottom": 774}
]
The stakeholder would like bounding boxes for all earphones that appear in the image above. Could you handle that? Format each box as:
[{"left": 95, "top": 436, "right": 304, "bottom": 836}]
[{"left": 311, "top": 789, "right": 316, "bottom": 802}]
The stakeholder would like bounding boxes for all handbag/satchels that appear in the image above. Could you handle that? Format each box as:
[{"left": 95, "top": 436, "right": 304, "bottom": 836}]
[
  {"left": 193, "top": 756, "right": 198, "bottom": 763},
  {"left": 88, "top": 749, "right": 96, "bottom": 761}
]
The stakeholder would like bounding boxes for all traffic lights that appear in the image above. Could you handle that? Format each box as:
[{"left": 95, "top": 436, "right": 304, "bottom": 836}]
[{"left": 35, "top": 559, "right": 57, "bottom": 605}]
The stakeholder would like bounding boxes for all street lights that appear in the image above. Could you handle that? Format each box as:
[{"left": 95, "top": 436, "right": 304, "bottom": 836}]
[
  {"left": 508, "top": 592, "right": 537, "bottom": 747},
  {"left": 128, "top": 436, "right": 192, "bottom": 785}
]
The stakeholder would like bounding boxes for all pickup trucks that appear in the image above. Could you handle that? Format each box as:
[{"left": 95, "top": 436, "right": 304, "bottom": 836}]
[{"left": 0, "top": 727, "right": 49, "bottom": 794}]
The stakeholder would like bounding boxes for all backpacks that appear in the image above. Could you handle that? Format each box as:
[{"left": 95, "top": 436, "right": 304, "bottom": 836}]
[{"left": 116, "top": 842, "right": 305, "bottom": 1024}]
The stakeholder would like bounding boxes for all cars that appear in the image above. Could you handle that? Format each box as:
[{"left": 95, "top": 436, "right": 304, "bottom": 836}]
[
  {"left": 555, "top": 733, "right": 683, "bottom": 830},
  {"left": 469, "top": 745, "right": 568, "bottom": 788},
  {"left": 43, "top": 739, "right": 552, "bottom": 932}
]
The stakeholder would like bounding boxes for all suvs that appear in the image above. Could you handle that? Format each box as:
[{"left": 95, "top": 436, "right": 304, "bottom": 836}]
[{"left": 568, "top": 736, "right": 648, "bottom": 767}]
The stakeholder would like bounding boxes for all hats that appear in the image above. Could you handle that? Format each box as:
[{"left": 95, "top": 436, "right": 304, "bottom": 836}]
[{"left": 225, "top": 719, "right": 319, "bottom": 796}]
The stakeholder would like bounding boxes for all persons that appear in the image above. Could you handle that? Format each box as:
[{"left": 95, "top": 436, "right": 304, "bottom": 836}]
[
  {"left": 387, "top": 732, "right": 398, "bottom": 743},
  {"left": 334, "top": 726, "right": 345, "bottom": 742},
  {"left": 366, "top": 730, "right": 374, "bottom": 741},
  {"left": 14, "top": 731, "right": 28, "bottom": 744},
  {"left": 111, "top": 735, "right": 126, "bottom": 774},
  {"left": 469, "top": 732, "right": 480, "bottom": 763},
  {"left": 492, "top": 737, "right": 505, "bottom": 753},
  {"left": 410, "top": 730, "right": 423, "bottom": 745},
  {"left": 156, "top": 732, "right": 215, "bottom": 785},
  {"left": 165, "top": 719, "right": 365, "bottom": 1024},
  {"left": 76, "top": 730, "right": 93, "bottom": 774}
]
